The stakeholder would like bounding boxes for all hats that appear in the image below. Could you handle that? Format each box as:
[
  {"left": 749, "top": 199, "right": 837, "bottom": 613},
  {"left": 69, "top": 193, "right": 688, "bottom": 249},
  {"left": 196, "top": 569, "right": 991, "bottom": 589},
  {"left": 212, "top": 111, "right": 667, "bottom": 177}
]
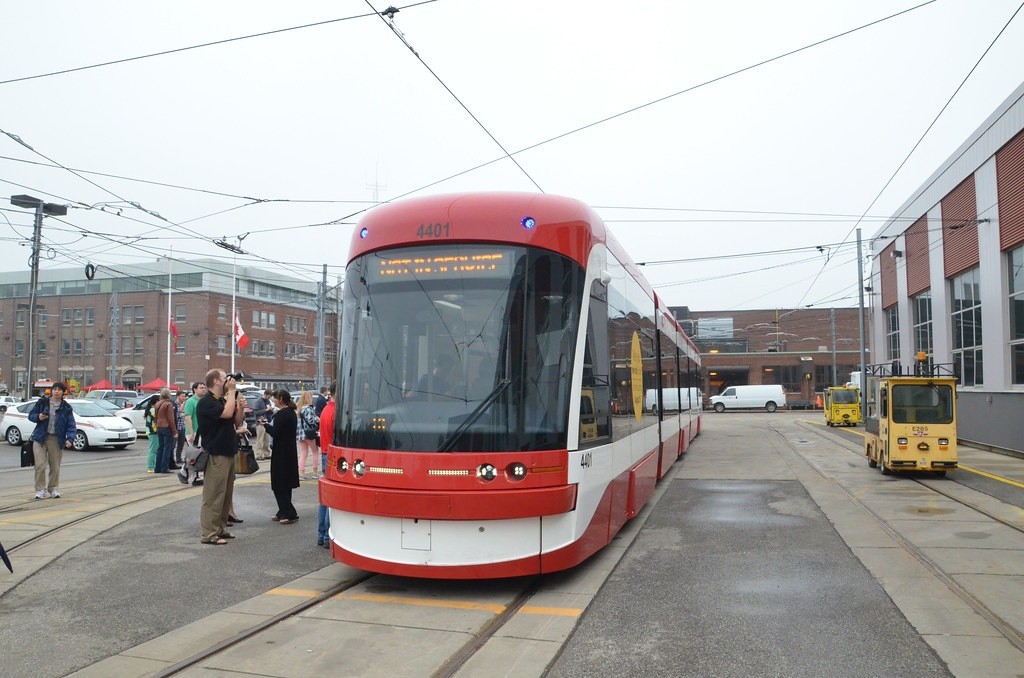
[{"left": 51, "top": 382, "right": 67, "bottom": 392}]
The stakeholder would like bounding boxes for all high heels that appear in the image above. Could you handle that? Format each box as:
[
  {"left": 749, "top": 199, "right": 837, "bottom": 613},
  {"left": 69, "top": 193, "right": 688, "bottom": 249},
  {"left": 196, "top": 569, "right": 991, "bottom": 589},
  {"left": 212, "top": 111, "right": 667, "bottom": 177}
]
[{"left": 226, "top": 514, "right": 243, "bottom": 526}]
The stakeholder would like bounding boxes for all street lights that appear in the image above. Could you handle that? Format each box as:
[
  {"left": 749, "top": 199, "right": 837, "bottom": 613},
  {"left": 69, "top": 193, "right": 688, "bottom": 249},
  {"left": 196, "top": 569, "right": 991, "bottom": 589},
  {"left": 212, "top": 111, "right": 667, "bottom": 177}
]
[{"left": 11, "top": 194, "right": 68, "bottom": 401}]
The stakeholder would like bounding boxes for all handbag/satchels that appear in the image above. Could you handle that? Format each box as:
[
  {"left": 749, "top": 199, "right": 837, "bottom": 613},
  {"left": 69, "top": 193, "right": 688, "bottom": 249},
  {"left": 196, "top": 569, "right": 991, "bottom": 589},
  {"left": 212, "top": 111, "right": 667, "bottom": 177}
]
[
  {"left": 21, "top": 441, "right": 34, "bottom": 467},
  {"left": 304, "top": 427, "right": 318, "bottom": 439},
  {"left": 236, "top": 432, "right": 259, "bottom": 474},
  {"left": 184, "top": 444, "right": 208, "bottom": 471}
]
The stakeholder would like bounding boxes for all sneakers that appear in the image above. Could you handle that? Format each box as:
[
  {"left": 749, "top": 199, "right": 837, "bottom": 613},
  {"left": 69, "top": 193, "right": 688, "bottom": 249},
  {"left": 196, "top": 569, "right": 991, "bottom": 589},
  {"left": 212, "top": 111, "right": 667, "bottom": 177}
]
[
  {"left": 177, "top": 472, "right": 205, "bottom": 486},
  {"left": 34, "top": 491, "right": 45, "bottom": 499},
  {"left": 49, "top": 490, "right": 61, "bottom": 498}
]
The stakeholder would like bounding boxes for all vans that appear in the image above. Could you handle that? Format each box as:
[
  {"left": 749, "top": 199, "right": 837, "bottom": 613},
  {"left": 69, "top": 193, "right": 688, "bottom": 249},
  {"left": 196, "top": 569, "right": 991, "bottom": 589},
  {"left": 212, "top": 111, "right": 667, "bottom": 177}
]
[{"left": 709, "top": 384, "right": 788, "bottom": 412}]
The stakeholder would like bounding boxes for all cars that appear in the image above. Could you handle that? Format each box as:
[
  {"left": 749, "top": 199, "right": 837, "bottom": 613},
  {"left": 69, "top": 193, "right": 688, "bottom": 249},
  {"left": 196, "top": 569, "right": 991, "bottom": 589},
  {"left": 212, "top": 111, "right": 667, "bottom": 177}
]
[
  {"left": 234, "top": 383, "right": 323, "bottom": 437},
  {"left": 1, "top": 390, "right": 194, "bottom": 451}
]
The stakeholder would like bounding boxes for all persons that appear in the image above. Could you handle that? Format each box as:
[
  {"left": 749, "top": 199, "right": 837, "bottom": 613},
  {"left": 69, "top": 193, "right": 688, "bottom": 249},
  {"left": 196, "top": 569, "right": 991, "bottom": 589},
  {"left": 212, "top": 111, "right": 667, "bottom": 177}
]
[
  {"left": 297, "top": 391, "right": 320, "bottom": 481},
  {"left": 177, "top": 382, "right": 205, "bottom": 487},
  {"left": 173, "top": 391, "right": 185, "bottom": 464},
  {"left": 258, "top": 389, "right": 300, "bottom": 523},
  {"left": 27, "top": 382, "right": 76, "bottom": 500},
  {"left": 317, "top": 379, "right": 337, "bottom": 550},
  {"left": 405, "top": 354, "right": 459, "bottom": 400},
  {"left": 155, "top": 387, "right": 179, "bottom": 473},
  {"left": 143, "top": 395, "right": 160, "bottom": 472},
  {"left": 254, "top": 390, "right": 275, "bottom": 461},
  {"left": 314, "top": 387, "right": 328, "bottom": 418},
  {"left": 196, "top": 367, "right": 248, "bottom": 544},
  {"left": 227, "top": 390, "right": 252, "bottom": 527}
]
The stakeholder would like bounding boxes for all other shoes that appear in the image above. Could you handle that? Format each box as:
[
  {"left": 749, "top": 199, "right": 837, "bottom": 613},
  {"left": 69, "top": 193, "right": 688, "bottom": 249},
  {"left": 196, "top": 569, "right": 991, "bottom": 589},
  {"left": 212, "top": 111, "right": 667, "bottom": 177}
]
[
  {"left": 255, "top": 456, "right": 271, "bottom": 461},
  {"left": 299, "top": 474, "right": 322, "bottom": 481},
  {"left": 270, "top": 445, "right": 274, "bottom": 449},
  {"left": 148, "top": 459, "right": 185, "bottom": 473},
  {"left": 272, "top": 514, "right": 300, "bottom": 524},
  {"left": 316, "top": 541, "right": 330, "bottom": 549}
]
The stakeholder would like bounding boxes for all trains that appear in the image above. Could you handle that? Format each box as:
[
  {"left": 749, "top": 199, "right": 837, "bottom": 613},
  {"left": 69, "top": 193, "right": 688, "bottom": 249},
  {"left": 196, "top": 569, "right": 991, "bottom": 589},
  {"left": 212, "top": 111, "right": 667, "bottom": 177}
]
[{"left": 320, "top": 190, "right": 704, "bottom": 582}]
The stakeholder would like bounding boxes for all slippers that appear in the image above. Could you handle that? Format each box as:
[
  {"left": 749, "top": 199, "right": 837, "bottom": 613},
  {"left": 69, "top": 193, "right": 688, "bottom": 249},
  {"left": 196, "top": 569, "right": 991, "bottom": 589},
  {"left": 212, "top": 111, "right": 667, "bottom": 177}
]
[{"left": 200, "top": 530, "right": 235, "bottom": 545}]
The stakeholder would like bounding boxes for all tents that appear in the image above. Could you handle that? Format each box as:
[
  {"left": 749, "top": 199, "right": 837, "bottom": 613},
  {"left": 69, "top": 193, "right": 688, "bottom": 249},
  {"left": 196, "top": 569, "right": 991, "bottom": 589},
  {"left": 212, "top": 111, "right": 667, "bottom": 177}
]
[
  {"left": 82, "top": 379, "right": 127, "bottom": 391},
  {"left": 136, "top": 377, "right": 181, "bottom": 391}
]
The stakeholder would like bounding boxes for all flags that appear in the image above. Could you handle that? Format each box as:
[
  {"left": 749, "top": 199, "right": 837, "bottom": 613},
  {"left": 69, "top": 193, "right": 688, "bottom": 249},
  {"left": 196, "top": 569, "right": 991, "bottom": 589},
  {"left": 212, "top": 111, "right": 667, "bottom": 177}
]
[
  {"left": 235, "top": 310, "right": 249, "bottom": 349},
  {"left": 170, "top": 314, "right": 178, "bottom": 352}
]
